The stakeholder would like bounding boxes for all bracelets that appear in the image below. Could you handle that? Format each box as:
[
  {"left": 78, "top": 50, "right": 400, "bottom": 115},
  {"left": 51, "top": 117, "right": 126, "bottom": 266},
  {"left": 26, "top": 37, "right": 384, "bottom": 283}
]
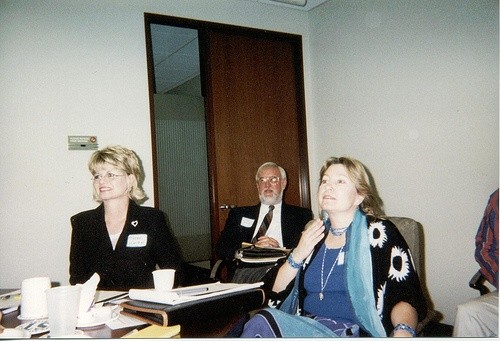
[
  {"left": 393, "top": 323, "right": 416, "bottom": 336},
  {"left": 287, "top": 249, "right": 302, "bottom": 268}
]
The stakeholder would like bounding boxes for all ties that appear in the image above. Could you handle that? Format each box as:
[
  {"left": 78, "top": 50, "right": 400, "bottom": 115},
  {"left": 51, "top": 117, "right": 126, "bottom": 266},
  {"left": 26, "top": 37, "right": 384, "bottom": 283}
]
[{"left": 251, "top": 205, "right": 275, "bottom": 244}]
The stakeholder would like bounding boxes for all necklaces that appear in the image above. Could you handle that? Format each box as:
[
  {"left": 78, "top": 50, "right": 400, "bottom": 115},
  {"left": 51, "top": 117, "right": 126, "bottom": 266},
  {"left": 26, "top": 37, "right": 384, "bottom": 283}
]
[{"left": 318, "top": 227, "right": 348, "bottom": 300}]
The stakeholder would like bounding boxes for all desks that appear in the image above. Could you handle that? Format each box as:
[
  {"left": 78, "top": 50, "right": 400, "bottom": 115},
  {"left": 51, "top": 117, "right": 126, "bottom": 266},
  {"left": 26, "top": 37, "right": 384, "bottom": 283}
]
[{"left": 0, "top": 288, "right": 249, "bottom": 338}]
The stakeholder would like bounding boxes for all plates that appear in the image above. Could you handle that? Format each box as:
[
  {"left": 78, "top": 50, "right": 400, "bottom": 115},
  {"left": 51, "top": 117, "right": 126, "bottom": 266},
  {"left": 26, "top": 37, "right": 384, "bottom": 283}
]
[
  {"left": 0, "top": 329, "right": 32, "bottom": 339},
  {"left": 17, "top": 314, "right": 48, "bottom": 321},
  {"left": 14, "top": 321, "right": 52, "bottom": 334},
  {"left": 77, "top": 321, "right": 106, "bottom": 330}
]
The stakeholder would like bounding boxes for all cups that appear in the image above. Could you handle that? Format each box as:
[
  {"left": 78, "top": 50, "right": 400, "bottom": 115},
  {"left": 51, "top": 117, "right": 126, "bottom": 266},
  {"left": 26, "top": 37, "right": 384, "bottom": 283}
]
[
  {"left": 47, "top": 286, "right": 79, "bottom": 338},
  {"left": 21, "top": 277, "right": 52, "bottom": 317},
  {"left": 91, "top": 306, "right": 119, "bottom": 323},
  {"left": 152, "top": 269, "right": 176, "bottom": 294}
]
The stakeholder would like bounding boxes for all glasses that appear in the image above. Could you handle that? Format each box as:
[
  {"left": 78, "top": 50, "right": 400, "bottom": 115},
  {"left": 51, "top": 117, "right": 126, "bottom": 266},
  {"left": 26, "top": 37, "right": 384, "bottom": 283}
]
[
  {"left": 90, "top": 173, "right": 124, "bottom": 181},
  {"left": 257, "top": 177, "right": 281, "bottom": 184}
]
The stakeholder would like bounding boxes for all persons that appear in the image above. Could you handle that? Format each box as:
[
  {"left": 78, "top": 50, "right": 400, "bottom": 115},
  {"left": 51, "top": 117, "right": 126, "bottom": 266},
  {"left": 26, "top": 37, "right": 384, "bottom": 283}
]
[
  {"left": 70, "top": 145, "right": 186, "bottom": 292},
  {"left": 241, "top": 157, "right": 426, "bottom": 338},
  {"left": 452, "top": 188, "right": 498, "bottom": 338},
  {"left": 217, "top": 161, "right": 313, "bottom": 310}
]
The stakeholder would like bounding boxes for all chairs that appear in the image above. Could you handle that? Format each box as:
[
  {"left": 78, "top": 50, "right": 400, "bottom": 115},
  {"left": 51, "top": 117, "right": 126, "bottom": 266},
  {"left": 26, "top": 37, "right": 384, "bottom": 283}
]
[{"left": 384, "top": 216, "right": 490, "bottom": 338}]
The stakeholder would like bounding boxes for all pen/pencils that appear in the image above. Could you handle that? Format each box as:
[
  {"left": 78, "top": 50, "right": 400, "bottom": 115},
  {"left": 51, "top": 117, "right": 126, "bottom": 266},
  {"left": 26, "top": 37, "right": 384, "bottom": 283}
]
[{"left": 181, "top": 287, "right": 209, "bottom": 294}]
